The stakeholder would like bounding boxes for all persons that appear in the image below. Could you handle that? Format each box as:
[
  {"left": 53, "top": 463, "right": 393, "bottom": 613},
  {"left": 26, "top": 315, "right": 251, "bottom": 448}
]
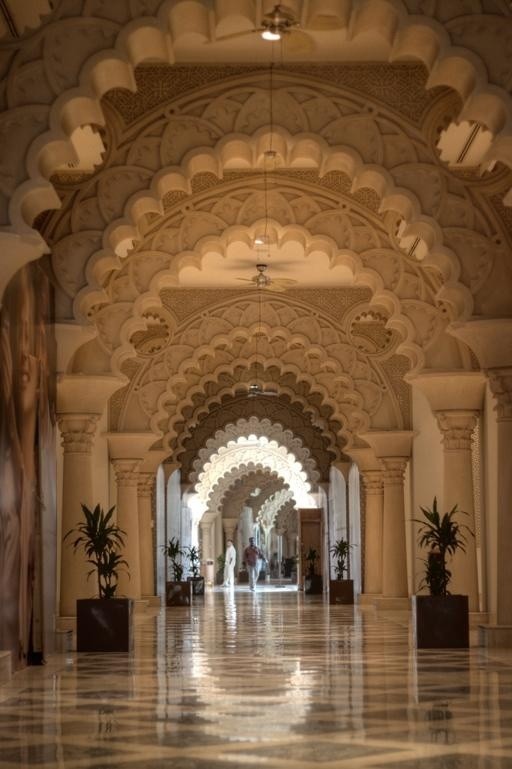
[
  {"left": 243, "top": 536, "right": 267, "bottom": 591},
  {"left": 220, "top": 540, "right": 236, "bottom": 587}
]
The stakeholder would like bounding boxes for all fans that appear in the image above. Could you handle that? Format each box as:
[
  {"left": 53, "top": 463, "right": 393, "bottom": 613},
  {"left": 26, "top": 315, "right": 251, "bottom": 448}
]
[
  {"left": 202, "top": 5, "right": 343, "bottom": 55},
  {"left": 247, "top": 385, "right": 277, "bottom": 399},
  {"left": 235, "top": 266, "right": 296, "bottom": 293}
]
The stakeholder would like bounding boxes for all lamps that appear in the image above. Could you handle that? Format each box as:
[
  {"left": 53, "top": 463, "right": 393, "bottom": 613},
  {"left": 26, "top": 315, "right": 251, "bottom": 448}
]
[{"left": 261, "top": 23, "right": 283, "bottom": 42}]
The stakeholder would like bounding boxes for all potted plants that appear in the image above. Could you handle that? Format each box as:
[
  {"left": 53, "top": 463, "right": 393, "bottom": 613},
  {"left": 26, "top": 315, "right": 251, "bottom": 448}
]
[
  {"left": 64, "top": 500, "right": 135, "bottom": 653},
  {"left": 328, "top": 536, "right": 359, "bottom": 604},
  {"left": 215, "top": 554, "right": 225, "bottom": 584},
  {"left": 160, "top": 535, "right": 194, "bottom": 607},
  {"left": 303, "top": 546, "right": 323, "bottom": 594},
  {"left": 404, "top": 496, "right": 476, "bottom": 650},
  {"left": 182, "top": 545, "right": 205, "bottom": 595},
  {"left": 290, "top": 555, "right": 299, "bottom": 583}
]
